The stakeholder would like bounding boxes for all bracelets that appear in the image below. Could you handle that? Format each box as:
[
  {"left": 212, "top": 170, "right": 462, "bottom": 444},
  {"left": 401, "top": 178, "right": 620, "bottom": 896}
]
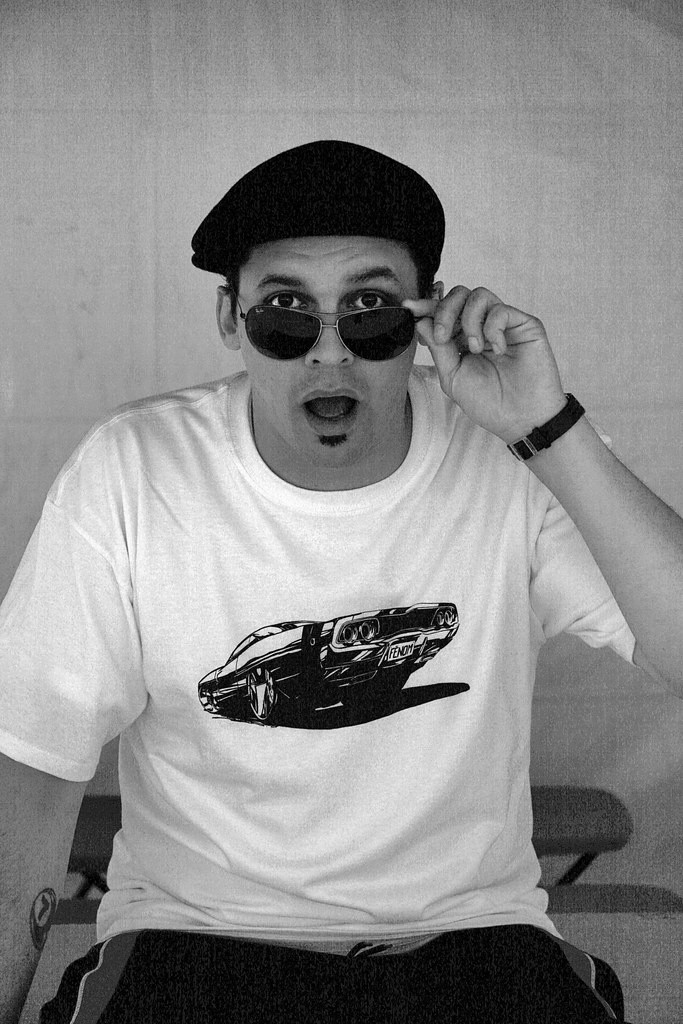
[{"left": 506, "top": 392, "right": 586, "bottom": 463}]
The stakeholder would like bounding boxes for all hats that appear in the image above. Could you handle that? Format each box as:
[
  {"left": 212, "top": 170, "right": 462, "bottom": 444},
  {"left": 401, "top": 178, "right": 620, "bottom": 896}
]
[{"left": 189, "top": 139, "right": 446, "bottom": 285}]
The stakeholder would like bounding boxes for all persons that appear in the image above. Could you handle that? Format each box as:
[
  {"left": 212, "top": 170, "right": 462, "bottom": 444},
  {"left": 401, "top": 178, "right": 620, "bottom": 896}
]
[{"left": 1, "top": 138, "right": 683, "bottom": 1024}]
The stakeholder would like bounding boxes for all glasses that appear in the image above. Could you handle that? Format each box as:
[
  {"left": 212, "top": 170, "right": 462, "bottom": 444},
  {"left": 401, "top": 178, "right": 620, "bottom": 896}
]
[{"left": 228, "top": 277, "right": 434, "bottom": 364}]
